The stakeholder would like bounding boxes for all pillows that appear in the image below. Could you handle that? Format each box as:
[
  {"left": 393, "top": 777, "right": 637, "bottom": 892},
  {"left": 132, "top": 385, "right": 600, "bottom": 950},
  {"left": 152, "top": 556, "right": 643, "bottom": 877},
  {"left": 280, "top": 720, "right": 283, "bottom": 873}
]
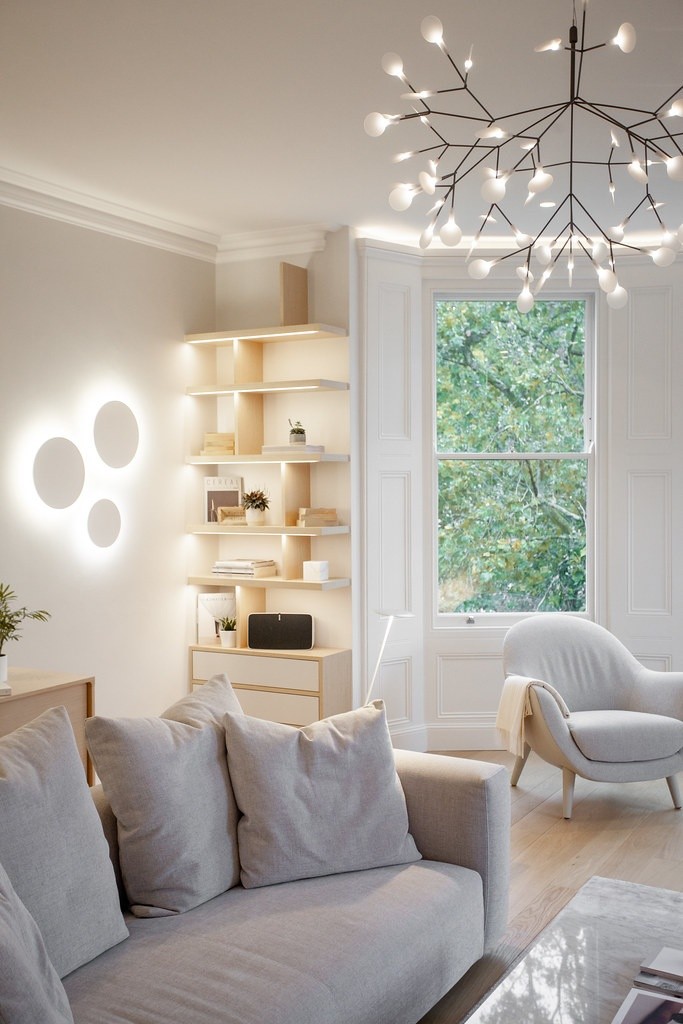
[
  {"left": 0, "top": 704, "right": 129, "bottom": 978},
  {"left": 84, "top": 674, "right": 250, "bottom": 919},
  {"left": 221, "top": 697, "right": 422, "bottom": 887},
  {"left": 0, "top": 863, "right": 73, "bottom": 1023}
]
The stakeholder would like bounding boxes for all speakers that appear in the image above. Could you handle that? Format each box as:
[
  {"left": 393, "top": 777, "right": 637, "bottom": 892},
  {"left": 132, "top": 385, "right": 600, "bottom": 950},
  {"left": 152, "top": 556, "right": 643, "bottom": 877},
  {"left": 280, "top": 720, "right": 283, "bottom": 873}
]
[{"left": 247, "top": 613, "right": 314, "bottom": 650}]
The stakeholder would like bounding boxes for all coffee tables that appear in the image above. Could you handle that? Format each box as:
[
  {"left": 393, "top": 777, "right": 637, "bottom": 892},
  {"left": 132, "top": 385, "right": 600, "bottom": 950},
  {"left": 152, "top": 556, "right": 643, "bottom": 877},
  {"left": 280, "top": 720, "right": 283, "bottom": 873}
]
[{"left": 465, "top": 873, "right": 683, "bottom": 1024}]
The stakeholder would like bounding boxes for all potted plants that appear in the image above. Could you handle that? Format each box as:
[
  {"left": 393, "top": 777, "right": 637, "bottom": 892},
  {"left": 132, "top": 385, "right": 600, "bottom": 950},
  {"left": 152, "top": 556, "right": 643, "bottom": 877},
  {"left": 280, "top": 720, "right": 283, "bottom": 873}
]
[
  {"left": 288, "top": 417, "right": 307, "bottom": 445},
  {"left": 242, "top": 488, "right": 270, "bottom": 526},
  {"left": 0, "top": 582, "right": 52, "bottom": 683},
  {"left": 215, "top": 615, "right": 237, "bottom": 649}
]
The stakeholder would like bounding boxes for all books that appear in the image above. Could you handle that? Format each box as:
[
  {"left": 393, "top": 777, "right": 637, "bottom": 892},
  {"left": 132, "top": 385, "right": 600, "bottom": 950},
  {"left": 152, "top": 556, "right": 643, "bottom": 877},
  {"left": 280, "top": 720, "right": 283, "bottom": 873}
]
[
  {"left": 611, "top": 946, "right": 683, "bottom": 1024},
  {"left": 204, "top": 476, "right": 241, "bottom": 523},
  {"left": 0, "top": 683, "right": 11, "bottom": 695},
  {"left": 211, "top": 559, "right": 274, "bottom": 576}
]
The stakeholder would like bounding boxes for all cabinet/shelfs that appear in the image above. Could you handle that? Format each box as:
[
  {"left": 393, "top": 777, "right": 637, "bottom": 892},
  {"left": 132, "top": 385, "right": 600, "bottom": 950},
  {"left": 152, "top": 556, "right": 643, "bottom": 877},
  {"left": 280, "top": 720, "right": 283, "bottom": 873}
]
[{"left": 185, "top": 322, "right": 354, "bottom": 727}]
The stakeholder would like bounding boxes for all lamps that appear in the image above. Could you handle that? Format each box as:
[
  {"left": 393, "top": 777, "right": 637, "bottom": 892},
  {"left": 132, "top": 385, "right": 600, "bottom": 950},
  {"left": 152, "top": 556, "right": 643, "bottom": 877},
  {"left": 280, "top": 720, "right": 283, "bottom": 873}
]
[{"left": 365, "top": 0, "right": 683, "bottom": 313}]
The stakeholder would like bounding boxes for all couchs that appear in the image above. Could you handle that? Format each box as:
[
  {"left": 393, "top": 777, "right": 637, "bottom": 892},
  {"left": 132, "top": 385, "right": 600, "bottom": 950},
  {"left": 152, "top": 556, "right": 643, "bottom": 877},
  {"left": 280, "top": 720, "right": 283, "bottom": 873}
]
[{"left": 0, "top": 749, "right": 513, "bottom": 1024}]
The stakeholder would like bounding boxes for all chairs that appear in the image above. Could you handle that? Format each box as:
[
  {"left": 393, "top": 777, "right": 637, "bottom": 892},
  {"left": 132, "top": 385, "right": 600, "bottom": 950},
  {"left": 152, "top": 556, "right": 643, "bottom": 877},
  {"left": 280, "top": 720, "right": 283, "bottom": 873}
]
[{"left": 500, "top": 615, "right": 682, "bottom": 821}]
